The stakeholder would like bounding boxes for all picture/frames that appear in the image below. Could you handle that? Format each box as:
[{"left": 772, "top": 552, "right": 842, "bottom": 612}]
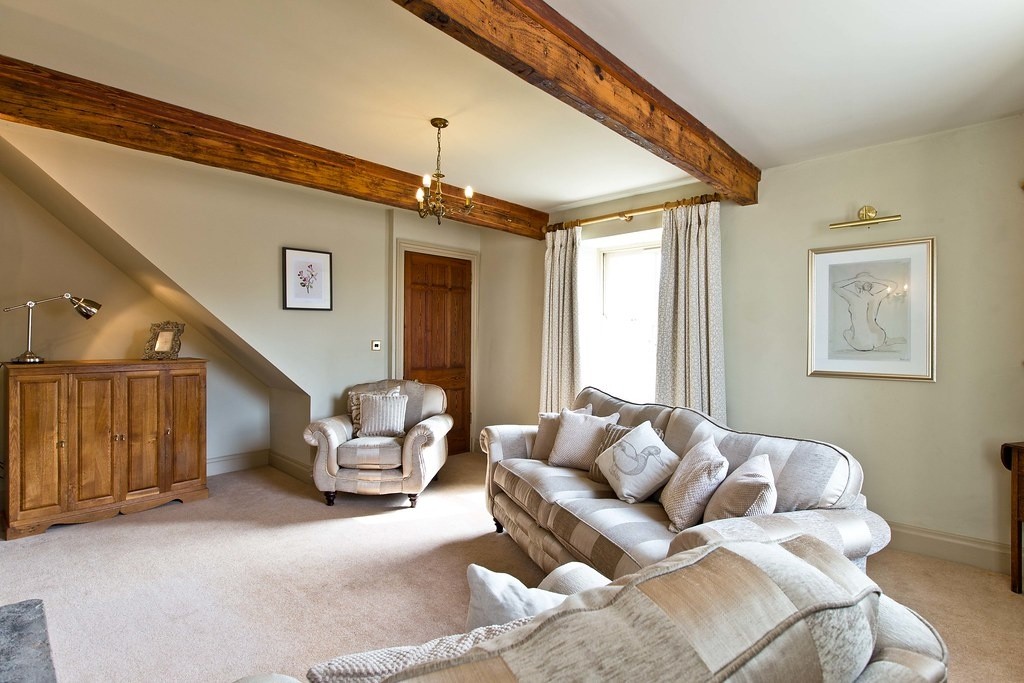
[
  {"left": 806, "top": 236, "right": 938, "bottom": 383},
  {"left": 282, "top": 247, "right": 333, "bottom": 310},
  {"left": 140, "top": 319, "right": 185, "bottom": 359}
]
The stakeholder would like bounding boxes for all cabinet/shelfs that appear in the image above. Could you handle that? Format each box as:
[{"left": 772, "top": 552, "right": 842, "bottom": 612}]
[{"left": 0, "top": 357, "right": 210, "bottom": 541}]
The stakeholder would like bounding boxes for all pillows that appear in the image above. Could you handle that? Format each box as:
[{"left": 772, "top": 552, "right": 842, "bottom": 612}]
[
  {"left": 346, "top": 378, "right": 447, "bottom": 435},
  {"left": 530, "top": 404, "right": 593, "bottom": 459},
  {"left": 548, "top": 409, "right": 620, "bottom": 471},
  {"left": 378, "top": 533, "right": 882, "bottom": 683},
  {"left": 703, "top": 454, "right": 778, "bottom": 523},
  {"left": 595, "top": 420, "right": 679, "bottom": 505},
  {"left": 348, "top": 386, "right": 400, "bottom": 438},
  {"left": 570, "top": 386, "right": 673, "bottom": 432},
  {"left": 357, "top": 395, "right": 408, "bottom": 438},
  {"left": 588, "top": 423, "right": 664, "bottom": 485},
  {"left": 465, "top": 564, "right": 569, "bottom": 634},
  {"left": 659, "top": 434, "right": 729, "bottom": 534},
  {"left": 662, "top": 405, "right": 865, "bottom": 512}
]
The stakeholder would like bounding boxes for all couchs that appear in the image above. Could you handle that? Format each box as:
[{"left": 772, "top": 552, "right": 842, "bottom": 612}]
[
  {"left": 302, "top": 413, "right": 455, "bottom": 508},
  {"left": 479, "top": 424, "right": 892, "bottom": 582},
  {"left": 234, "top": 593, "right": 949, "bottom": 683}
]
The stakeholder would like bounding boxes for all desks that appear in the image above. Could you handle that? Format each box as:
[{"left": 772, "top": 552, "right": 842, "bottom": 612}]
[{"left": 1001, "top": 441, "right": 1024, "bottom": 594}]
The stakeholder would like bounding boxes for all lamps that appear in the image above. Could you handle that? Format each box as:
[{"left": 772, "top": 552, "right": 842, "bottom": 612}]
[
  {"left": 415, "top": 117, "right": 474, "bottom": 226},
  {"left": 3, "top": 292, "right": 102, "bottom": 363}
]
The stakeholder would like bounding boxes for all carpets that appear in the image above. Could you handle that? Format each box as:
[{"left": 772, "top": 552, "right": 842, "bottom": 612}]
[{"left": 0, "top": 598, "right": 57, "bottom": 683}]
